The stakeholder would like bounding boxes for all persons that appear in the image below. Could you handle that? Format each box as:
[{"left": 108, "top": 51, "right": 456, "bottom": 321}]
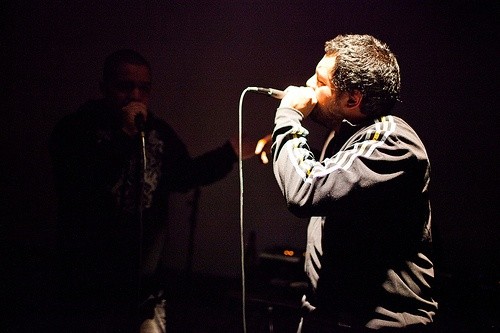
[
  {"left": 255, "top": 35, "right": 439, "bottom": 332},
  {"left": 52, "top": 46, "right": 257, "bottom": 270}
]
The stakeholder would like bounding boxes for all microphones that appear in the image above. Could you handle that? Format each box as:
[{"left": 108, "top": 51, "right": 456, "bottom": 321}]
[
  {"left": 135, "top": 113, "right": 153, "bottom": 137},
  {"left": 245, "top": 86, "right": 284, "bottom": 99}
]
